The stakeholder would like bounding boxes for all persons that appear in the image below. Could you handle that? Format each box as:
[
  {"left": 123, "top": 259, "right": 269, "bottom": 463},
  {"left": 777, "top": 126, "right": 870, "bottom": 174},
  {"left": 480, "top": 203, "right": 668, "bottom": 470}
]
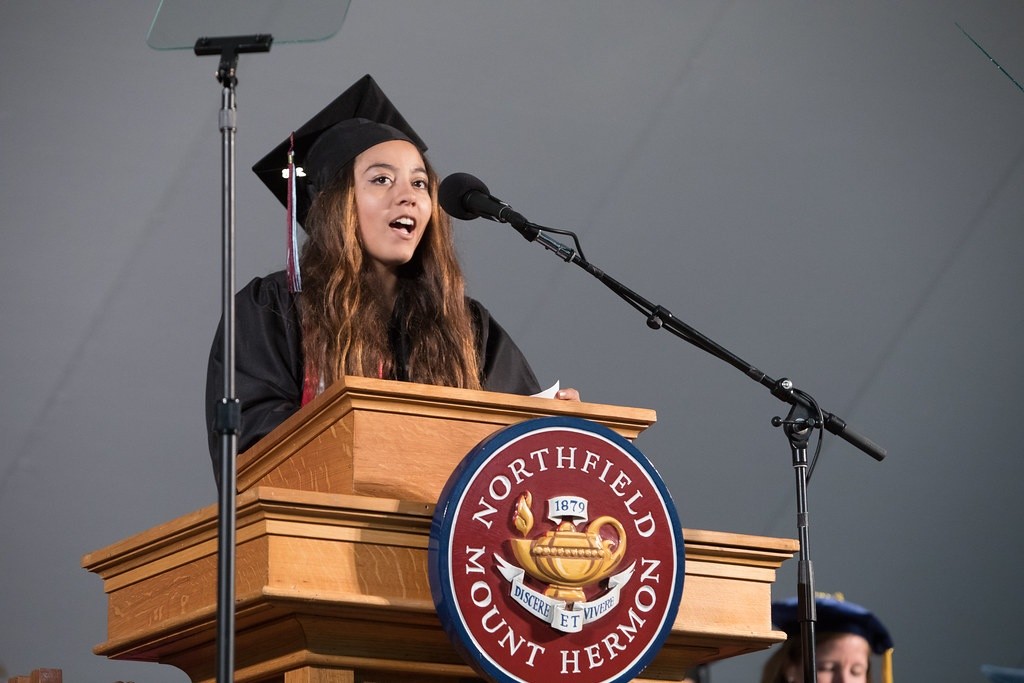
[
  {"left": 203, "top": 72, "right": 581, "bottom": 497},
  {"left": 761, "top": 590, "right": 895, "bottom": 683}
]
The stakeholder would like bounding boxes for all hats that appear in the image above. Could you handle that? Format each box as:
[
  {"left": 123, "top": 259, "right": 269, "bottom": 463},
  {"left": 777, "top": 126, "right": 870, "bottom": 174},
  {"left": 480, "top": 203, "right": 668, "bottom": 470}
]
[
  {"left": 771, "top": 593, "right": 894, "bottom": 683},
  {"left": 251, "top": 74, "right": 429, "bottom": 291}
]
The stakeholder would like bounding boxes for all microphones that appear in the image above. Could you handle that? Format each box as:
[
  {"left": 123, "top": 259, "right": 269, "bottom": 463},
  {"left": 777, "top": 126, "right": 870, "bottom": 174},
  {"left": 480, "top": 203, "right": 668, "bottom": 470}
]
[{"left": 437, "top": 172, "right": 540, "bottom": 243}]
